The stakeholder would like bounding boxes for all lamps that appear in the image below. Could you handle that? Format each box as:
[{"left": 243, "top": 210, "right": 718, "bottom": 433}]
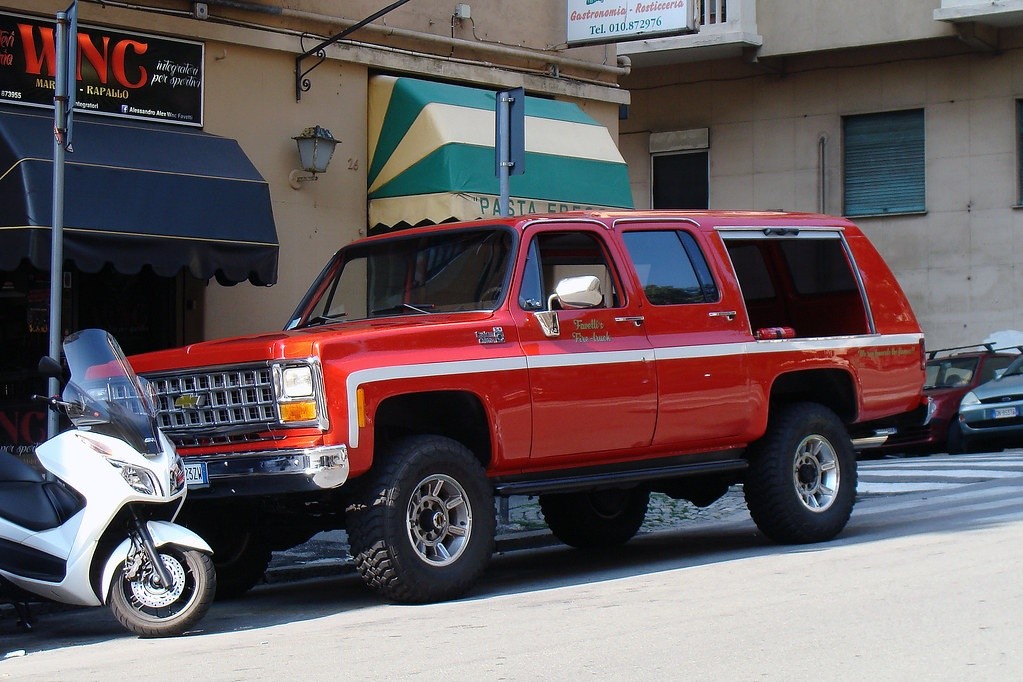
[{"left": 289, "top": 126, "right": 343, "bottom": 189}]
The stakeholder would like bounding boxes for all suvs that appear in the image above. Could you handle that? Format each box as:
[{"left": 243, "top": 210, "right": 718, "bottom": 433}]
[{"left": 80, "top": 207, "right": 927, "bottom": 603}]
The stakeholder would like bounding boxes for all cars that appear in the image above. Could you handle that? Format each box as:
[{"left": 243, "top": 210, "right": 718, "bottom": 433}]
[
  {"left": 958, "top": 353, "right": 1023, "bottom": 450},
  {"left": 862, "top": 342, "right": 1023, "bottom": 458}
]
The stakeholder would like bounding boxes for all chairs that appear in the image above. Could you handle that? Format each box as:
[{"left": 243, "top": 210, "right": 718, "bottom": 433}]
[
  {"left": 548, "top": 265, "right": 614, "bottom": 308},
  {"left": 945, "top": 374, "right": 962, "bottom": 384}
]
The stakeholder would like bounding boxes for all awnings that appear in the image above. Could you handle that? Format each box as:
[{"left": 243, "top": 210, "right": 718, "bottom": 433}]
[
  {"left": 0, "top": 104, "right": 280, "bottom": 288},
  {"left": 367, "top": 74, "right": 636, "bottom": 231}
]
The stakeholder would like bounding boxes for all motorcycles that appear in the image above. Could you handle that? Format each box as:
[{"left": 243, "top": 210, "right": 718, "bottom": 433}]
[{"left": 0, "top": 329, "right": 216, "bottom": 637}]
